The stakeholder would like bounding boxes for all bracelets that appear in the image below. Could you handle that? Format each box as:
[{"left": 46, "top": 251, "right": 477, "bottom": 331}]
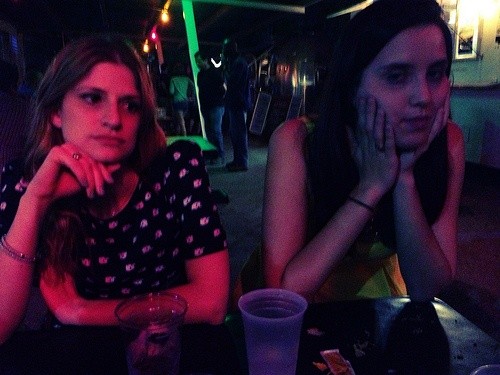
[
  {"left": 0, "top": 234, "right": 38, "bottom": 264},
  {"left": 345, "top": 194, "right": 376, "bottom": 214}
]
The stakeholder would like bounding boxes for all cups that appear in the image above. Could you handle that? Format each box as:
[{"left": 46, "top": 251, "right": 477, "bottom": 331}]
[
  {"left": 238, "top": 288, "right": 308, "bottom": 375},
  {"left": 114, "top": 292, "right": 187, "bottom": 375}
]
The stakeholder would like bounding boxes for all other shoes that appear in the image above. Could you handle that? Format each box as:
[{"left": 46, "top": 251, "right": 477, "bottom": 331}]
[{"left": 225, "top": 161, "right": 248, "bottom": 173}]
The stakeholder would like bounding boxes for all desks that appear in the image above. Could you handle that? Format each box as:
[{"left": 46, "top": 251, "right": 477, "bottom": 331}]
[{"left": 0, "top": 295, "right": 500, "bottom": 375}]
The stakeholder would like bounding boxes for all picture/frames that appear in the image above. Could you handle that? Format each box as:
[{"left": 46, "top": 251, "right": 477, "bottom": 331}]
[{"left": 452, "top": 0, "right": 484, "bottom": 63}]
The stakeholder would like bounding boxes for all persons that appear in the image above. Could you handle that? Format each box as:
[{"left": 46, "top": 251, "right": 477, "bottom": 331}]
[
  {"left": 222, "top": 38, "right": 254, "bottom": 171},
  {"left": 195, "top": 51, "right": 227, "bottom": 164},
  {"left": 0, "top": 34, "right": 230, "bottom": 347},
  {"left": 141, "top": 48, "right": 194, "bottom": 137},
  {"left": 230, "top": 0, "right": 465, "bottom": 314},
  {"left": 0, "top": 59, "right": 43, "bottom": 174}
]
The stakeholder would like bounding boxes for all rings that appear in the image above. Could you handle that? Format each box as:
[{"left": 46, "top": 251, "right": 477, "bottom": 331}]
[
  {"left": 375, "top": 143, "right": 385, "bottom": 152},
  {"left": 73, "top": 152, "right": 81, "bottom": 160}
]
